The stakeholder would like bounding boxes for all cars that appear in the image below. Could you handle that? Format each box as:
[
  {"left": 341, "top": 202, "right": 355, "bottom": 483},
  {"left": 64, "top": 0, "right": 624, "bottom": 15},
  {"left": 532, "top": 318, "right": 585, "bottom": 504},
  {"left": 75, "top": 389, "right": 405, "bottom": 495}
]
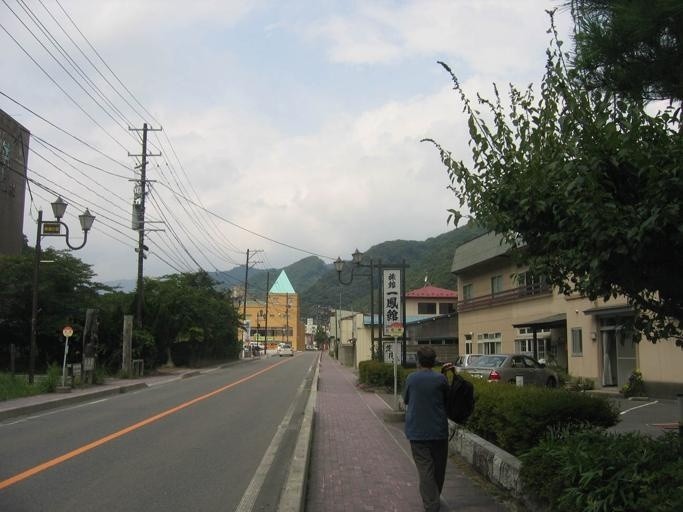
[
  {"left": 244, "top": 341, "right": 293, "bottom": 358},
  {"left": 400, "top": 351, "right": 559, "bottom": 389},
  {"left": 305, "top": 342, "right": 320, "bottom": 351}
]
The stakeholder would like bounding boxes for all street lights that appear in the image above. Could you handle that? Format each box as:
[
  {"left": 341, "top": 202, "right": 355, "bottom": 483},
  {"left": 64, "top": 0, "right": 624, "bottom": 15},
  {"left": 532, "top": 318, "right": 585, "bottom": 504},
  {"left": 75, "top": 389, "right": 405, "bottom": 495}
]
[
  {"left": 333, "top": 247, "right": 375, "bottom": 360},
  {"left": 319, "top": 310, "right": 337, "bottom": 357},
  {"left": 255, "top": 309, "right": 265, "bottom": 352},
  {"left": 28, "top": 192, "right": 97, "bottom": 386}
]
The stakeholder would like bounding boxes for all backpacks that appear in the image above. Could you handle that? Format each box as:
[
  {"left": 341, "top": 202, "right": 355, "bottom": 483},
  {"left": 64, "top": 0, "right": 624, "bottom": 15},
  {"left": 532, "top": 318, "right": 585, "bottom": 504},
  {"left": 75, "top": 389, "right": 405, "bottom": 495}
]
[{"left": 441, "top": 366, "right": 475, "bottom": 425}]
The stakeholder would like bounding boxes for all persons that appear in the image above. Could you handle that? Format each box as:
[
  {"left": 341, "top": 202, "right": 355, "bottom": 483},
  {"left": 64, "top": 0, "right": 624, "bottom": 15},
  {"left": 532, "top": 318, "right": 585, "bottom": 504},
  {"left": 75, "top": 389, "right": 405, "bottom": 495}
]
[{"left": 403, "top": 348, "right": 454, "bottom": 511}]
